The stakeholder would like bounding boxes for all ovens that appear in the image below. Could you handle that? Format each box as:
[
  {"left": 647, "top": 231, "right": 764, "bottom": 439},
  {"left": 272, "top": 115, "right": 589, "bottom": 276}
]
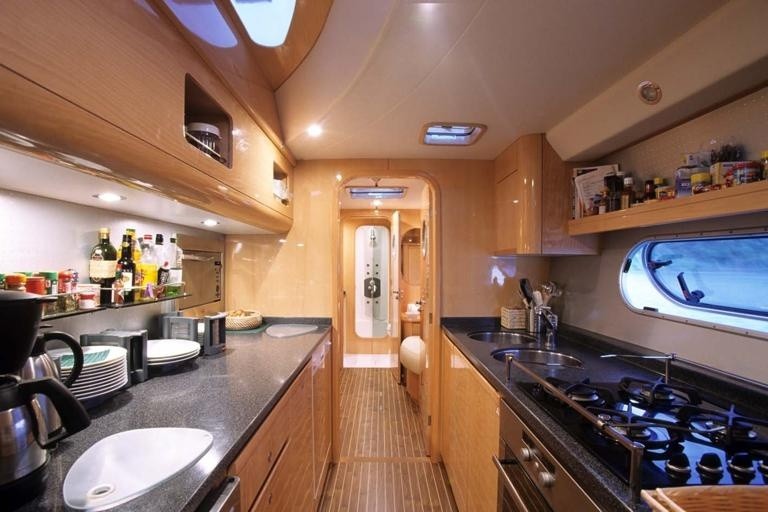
[{"left": 494, "top": 399, "right": 603, "bottom": 512}]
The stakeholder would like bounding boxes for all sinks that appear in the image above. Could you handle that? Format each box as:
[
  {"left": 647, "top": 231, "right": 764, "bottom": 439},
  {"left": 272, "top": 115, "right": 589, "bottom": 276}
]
[
  {"left": 492, "top": 349, "right": 583, "bottom": 367},
  {"left": 469, "top": 332, "right": 536, "bottom": 344}
]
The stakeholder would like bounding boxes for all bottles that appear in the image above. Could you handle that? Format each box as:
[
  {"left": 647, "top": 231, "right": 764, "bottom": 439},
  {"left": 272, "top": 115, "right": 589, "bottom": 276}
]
[
  {"left": 187, "top": 122, "right": 221, "bottom": 157},
  {"left": 536, "top": 305, "right": 559, "bottom": 338},
  {"left": 78, "top": 292, "right": 95, "bottom": 309},
  {"left": 597, "top": 171, "right": 666, "bottom": 217},
  {"left": 89, "top": 226, "right": 181, "bottom": 308},
  {"left": 0, "top": 269, "right": 78, "bottom": 313}
]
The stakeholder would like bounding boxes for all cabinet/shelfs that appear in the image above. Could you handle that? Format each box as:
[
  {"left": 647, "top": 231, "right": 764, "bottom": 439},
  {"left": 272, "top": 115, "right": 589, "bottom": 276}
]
[
  {"left": 314, "top": 333, "right": 337, "bottom": 496},
  {"left": 440, "top": 329, "right": 503, "bottom": 512},
  {"left": 201, "top": 472, "right": 242, "bottom": 511},
  {"left": 490, "top": 134, "right": 603, "bottom": 259},
  {"left": 225, "top": 357, "right": 318, "bottom": 512}
]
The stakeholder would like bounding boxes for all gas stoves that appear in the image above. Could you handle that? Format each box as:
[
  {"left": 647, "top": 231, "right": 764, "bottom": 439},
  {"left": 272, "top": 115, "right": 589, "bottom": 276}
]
[{"left": 512, "top": 378, "right": 767, "bottom": 490}]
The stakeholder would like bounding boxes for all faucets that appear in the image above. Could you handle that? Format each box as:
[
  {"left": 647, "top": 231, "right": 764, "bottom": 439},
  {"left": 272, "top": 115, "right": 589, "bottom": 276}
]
[{"left": 534, "top": 305, "right": 559, "bottom": 348}]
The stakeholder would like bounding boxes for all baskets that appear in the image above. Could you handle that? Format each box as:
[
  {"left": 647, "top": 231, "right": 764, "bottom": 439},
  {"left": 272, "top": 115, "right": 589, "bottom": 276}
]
[
  {"left": 501, "top": 306, "right": 526, "bottom": 330},
  {"left": 656, "top": 485, "right": 768, "bottom": 512},
  {"left": 640, "top": 489, "right": 672, "bottom": 512},
  {"left": 224, "top": 311, "right": 263, "bottom": 331}
]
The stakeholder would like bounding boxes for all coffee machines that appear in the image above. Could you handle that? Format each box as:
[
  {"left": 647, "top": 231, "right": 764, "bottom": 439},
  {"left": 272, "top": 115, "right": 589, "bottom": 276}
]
[{"left": 0, "top": 289, "right": 93, "bottom": 503}]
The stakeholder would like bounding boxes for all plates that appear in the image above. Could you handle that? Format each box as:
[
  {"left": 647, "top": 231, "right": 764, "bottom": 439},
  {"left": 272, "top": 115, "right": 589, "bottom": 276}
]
[
  {"left": 42, "top": 345, "right": 131, "bottom": 407},
  {"left": 146, "top": 338, "right": 202, "bottom": 368}
]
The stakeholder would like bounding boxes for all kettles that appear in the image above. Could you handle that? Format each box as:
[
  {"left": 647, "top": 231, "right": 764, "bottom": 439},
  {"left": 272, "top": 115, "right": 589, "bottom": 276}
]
[{"left": 19, "top": 324, "right": 86, "bottom": 444}]
